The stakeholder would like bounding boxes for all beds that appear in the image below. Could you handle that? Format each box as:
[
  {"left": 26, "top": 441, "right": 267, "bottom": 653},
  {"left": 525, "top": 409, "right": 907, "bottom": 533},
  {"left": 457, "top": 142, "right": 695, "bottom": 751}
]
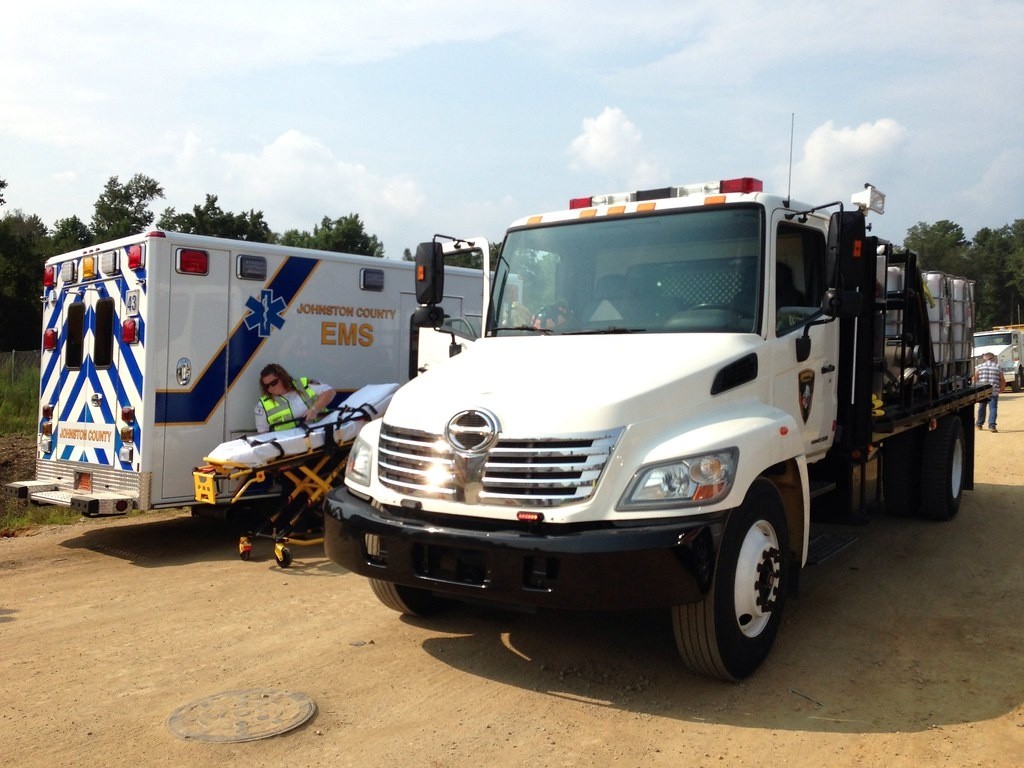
[{"left": 193, "top": 382, "right": 400, "bottom": 567}]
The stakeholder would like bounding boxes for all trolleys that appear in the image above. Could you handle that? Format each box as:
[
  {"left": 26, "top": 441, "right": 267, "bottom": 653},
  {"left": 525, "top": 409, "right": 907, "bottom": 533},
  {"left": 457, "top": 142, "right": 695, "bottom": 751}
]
[{"left": 190, "top": 403, "right": 382, "bottom": 569}]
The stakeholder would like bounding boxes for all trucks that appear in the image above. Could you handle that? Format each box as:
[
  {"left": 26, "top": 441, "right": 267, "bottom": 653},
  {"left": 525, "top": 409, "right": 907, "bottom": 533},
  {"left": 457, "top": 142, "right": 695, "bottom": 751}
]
[
  {"left": 971, "top": 330, "right": 1024, "bottom": 393},
  {"left": 321, "top": 178, "right": 997, "bottom": 682}
]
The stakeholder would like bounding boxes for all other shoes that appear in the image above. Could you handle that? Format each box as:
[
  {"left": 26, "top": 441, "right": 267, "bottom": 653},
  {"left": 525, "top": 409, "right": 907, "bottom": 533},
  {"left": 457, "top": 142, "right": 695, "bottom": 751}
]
[
  {"left": 989, "top": 427, "right": 998, "bottom": 433},
  {"left": 976, "top": 424, "right": 982, "bottom": 430}
]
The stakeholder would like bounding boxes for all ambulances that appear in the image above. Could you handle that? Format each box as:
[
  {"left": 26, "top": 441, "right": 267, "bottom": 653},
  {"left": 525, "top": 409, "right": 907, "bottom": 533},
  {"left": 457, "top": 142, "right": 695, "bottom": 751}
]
[{"left": 0, "top": 228, "right": 523, "bottom": 514}]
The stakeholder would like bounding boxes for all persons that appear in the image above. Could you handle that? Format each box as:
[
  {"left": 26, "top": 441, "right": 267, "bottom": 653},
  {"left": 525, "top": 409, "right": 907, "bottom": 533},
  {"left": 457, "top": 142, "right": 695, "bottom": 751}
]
[
  {"left": 254, "top": 364, "right": 337, "bottom": 435},
  {"left": 974, "top": 353, "right": 1006, "bottom": 432},
  {"left": 727, "top": 255, "right": 802, "bottom": 320}
]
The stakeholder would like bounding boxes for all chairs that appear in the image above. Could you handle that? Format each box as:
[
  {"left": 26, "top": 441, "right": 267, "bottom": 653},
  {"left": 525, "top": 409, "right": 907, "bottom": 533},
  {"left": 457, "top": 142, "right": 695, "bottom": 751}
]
[
  {"left": 730, "top": 263, "right": 806, "bottom": 319},
  {"left": 574, "top": 274, "right": 649, "bottom": 330}
]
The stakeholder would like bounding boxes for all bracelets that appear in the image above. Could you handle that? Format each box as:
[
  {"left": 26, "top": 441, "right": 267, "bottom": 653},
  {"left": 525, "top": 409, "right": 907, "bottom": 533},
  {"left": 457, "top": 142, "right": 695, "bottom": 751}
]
[{"left": 310, "top": 406, "right": 319, "bottom": 415}]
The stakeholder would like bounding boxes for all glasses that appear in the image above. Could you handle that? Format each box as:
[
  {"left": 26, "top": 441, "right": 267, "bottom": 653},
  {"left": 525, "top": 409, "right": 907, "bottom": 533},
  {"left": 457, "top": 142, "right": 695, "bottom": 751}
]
[{"left": 264, "top": 377, "right": 279, "bottom": 389}]
